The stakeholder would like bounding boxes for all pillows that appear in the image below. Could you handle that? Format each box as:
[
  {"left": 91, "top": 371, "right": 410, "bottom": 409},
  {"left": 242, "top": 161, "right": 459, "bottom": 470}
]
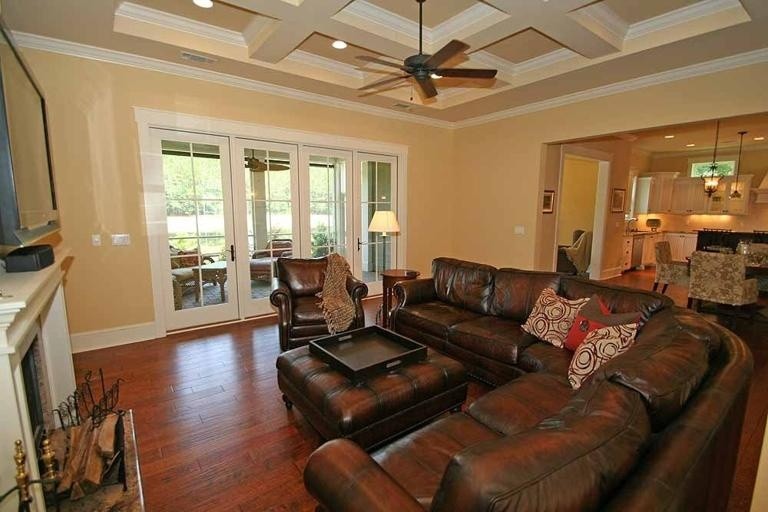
[
  {"left": 520, "top": 288, "right": 645, "bottom": 390},
  {"left": 178, "top": 246, "right": 200, "bottom": 255},
  {"left": 171, "top": 255, "right": 202, "bottom": 268}
]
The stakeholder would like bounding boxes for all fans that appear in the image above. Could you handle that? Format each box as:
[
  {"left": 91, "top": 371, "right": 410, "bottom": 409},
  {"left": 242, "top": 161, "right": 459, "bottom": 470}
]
[
  {"left": 354, "top": 0, "right": 498, "bottom": 99},
  {"left": 245, "top": 149, "right": 289, "bottom": 172}
]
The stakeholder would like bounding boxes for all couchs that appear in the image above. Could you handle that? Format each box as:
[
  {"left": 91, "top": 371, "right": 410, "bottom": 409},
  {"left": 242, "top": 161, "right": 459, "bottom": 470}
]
[{"left": 303, "top": 257, "right": 757, "bottom": 511}]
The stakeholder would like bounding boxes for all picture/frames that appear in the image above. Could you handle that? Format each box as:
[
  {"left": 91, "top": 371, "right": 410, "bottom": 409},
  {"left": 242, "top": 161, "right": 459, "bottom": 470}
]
[
  {"left": 611, "top": 188, "right": 626, "bottom": 213},
  {"left": 542, "top": 190, "right": 555, "bottom": 213}
]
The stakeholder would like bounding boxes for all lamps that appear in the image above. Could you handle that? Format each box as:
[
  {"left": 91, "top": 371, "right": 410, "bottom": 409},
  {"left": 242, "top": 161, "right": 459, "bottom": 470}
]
[
  {"left": 729, "top": 131, "right": 747, "bottom": 199},
  {"left": 700, "top": 120, "right": 725, "bottom": 197},
  {"left": 368, "top": 210, "right": 400, "bottom": 329}
]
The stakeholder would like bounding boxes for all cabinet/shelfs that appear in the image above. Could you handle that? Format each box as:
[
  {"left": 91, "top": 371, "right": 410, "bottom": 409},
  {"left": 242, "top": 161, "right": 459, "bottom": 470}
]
[
  {"left": 709, "top": 175, "right": 755, "bottom": 216},
  {"left": 641, "top": 234, "right": 665, "bottom": 265},
  {"left": 672, "top": 177, "right": 708, "bottom": 215},
  {"left": 667, "top": 233, "right": 698, "bottom": 263},
  {"left": 635, "top": 177, "right": 673, "bottom": 214},
  {"left": 622, "top": 235, "right": 643, "bottom": 270}
]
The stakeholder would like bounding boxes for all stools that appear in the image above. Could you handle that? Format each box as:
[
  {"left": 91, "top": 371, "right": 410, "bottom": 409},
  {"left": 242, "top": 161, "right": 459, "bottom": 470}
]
[{"left": 276, "top": 324, "right": 468, "bottom": 454}]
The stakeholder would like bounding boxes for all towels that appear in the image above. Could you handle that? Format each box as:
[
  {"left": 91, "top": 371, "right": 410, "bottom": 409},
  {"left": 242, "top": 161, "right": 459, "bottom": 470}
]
[{"left": 564, "top": 231, "right": 593, "bottom": 271}]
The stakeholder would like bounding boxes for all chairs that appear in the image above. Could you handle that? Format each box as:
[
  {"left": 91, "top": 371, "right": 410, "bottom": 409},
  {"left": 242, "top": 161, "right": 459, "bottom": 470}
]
[
  {"left": 558, "top": 230, "right": 584, "bottom": 247},
  {"left": 559, "top": 230, "right": 592, "bottom": 272},
  {"left": 652, "top": 241, "right": 690, "bottom": 294},
  {"left": 688, "top": 251, "right": 758, "bottom": 331},
  {"left": 270, "top": 255, "right": 367, "bottom": 351},
  {"left": 170, "top": 245, "right": 217, "bottom": 311},
  {"left": 250, "top": 239, "right": 293, "bottom": 282}
]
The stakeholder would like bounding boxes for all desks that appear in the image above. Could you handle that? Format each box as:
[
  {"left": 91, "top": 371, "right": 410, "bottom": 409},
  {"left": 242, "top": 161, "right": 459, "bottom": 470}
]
[
  {"left": 192, "top": 261, "right": 227, "bottom": 302},
  {"left": 381, "top": 269, "right": 420, "bottom": 329},
  {"left": 687, "top": 257, "right": 768, "bottom": 309}
]
